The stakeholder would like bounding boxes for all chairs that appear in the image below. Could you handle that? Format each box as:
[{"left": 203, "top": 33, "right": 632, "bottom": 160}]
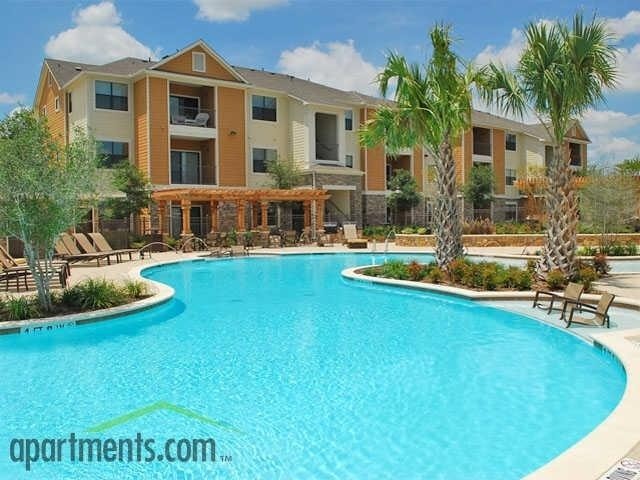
[
  {"left": 170, "top": 111, "right": 210, "bottom": 127},
  {"left": 532, "top": 279, "right": 615, "bottom": 328},
  {"left": 0, "top": 219, "right": 369, "bottom": 295}
]
